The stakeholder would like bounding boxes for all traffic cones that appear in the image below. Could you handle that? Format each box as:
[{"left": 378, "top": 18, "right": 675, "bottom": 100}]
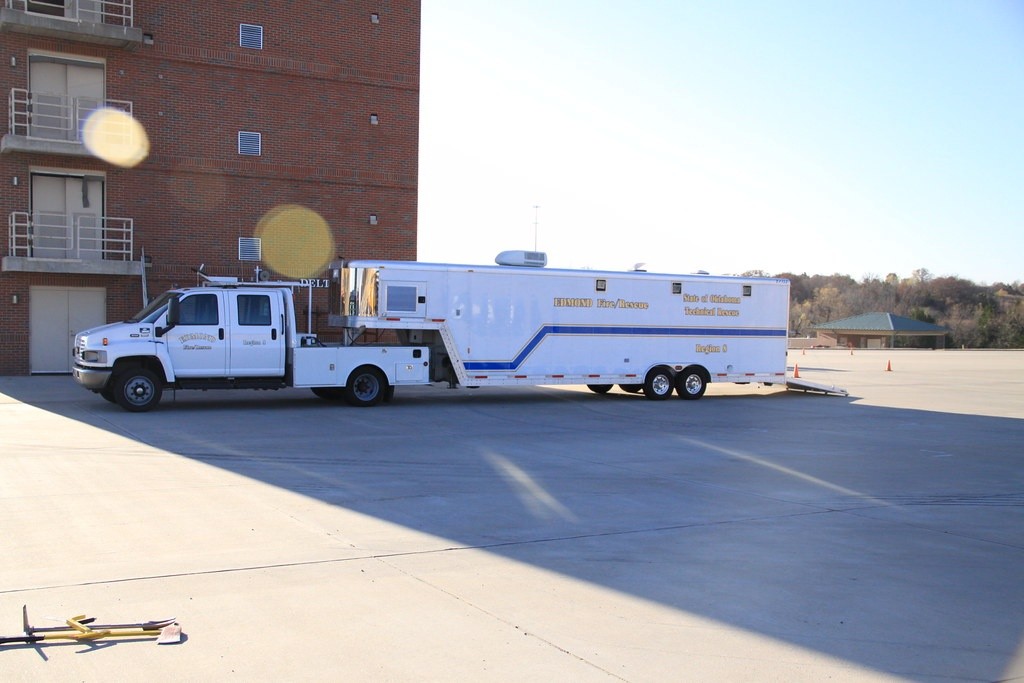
[
  {"left": 885, "top": 360, "right": 892, "bottom": 372},
  {"left": 802, "top": 347, "right": 806, "bottom": 357},
  {"left": 850, "top": 348, "right": 854, "bottom": 356},
  {"left": 793, "top": 363, "right": 801, "bottom": 378}
]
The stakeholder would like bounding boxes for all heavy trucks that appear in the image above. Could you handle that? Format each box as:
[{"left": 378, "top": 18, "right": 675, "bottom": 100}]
[{"left": 69, "top": 252, "right": 794, "bottom": 413}]
